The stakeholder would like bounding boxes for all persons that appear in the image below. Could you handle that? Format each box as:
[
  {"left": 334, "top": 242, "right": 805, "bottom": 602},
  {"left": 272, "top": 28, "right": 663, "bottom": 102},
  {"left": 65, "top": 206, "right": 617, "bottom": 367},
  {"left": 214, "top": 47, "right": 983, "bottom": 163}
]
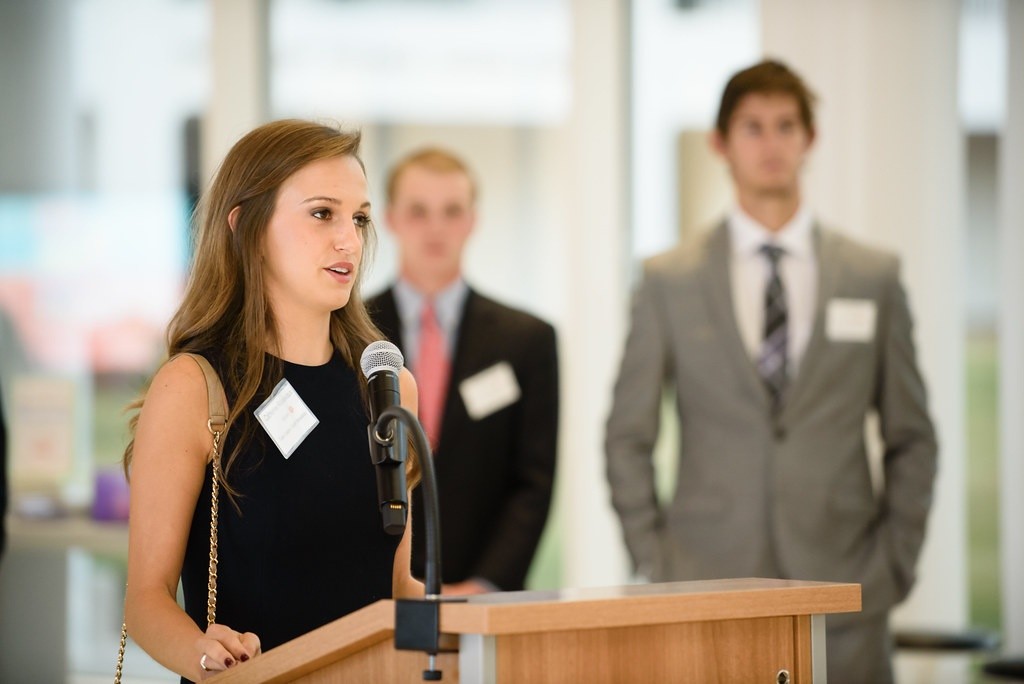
[
  {"left": 341, "top": 139, "right": 560, "bottom": 600},
  {"left": 120, "top": 119, "right": 434, "bottom": 682},
  {"left": 603, "top": 57, "right": 942, "bottom": 684}
]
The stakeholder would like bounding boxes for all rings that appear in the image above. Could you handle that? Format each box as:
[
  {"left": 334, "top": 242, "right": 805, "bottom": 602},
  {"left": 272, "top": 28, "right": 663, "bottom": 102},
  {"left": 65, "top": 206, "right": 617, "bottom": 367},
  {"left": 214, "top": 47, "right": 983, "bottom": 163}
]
[{"left": 199, "top": 653, "right": 212, "bottom": 671}]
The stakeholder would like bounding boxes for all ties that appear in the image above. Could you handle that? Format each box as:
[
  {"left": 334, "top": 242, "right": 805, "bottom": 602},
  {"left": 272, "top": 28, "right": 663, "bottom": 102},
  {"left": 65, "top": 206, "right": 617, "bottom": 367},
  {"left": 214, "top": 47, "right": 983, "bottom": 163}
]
[
  {"left": 412, "top": 306, "right": 450, "bottom": 457},
  {"left": 757, "top": 245, "right": 788, "bottom": 420}
]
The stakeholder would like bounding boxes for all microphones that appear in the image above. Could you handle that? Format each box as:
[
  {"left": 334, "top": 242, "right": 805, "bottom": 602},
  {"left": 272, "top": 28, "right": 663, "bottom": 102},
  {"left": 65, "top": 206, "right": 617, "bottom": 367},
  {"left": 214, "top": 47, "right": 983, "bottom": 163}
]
[{"left": 360, "top": 340, "right": 409, "bottom": 535}]
